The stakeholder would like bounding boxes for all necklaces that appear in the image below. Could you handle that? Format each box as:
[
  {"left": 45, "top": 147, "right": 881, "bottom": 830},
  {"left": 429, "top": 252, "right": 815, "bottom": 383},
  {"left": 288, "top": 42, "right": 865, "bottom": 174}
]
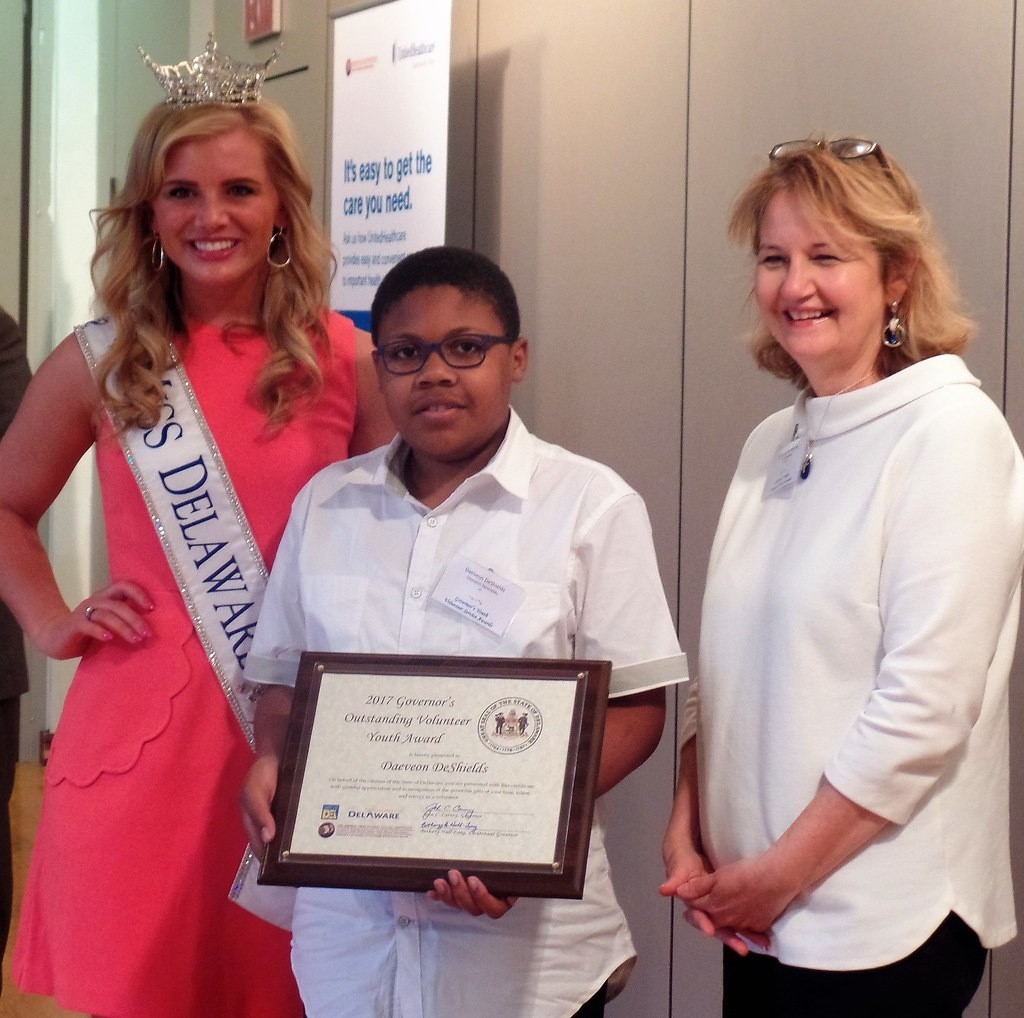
[{"left": 799, "top": 368, "right": 879, "bottom": 481}]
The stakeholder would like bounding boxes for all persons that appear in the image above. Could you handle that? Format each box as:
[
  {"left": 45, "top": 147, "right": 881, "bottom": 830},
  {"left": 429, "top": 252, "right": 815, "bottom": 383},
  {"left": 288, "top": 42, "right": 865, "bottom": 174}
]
[
  {"left": 234, "top": 247, "right": 691, "bottom": 1018},
  {"left": 0, "top": 308, "right": 34, "bottom": 994},
  {"left": 655, "top": 135, "right": 1024, "bottom": 1017},
  {"left": 0, "top": 87, "right": 404, "bottom": 1015}
]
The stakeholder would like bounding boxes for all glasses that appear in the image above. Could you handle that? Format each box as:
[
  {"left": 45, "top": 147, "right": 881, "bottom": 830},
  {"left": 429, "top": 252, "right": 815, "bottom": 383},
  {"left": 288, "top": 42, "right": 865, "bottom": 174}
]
[
  {"left": 768, "top": 138, "right": 893, "bottom": 181},
  {"left": 378, "top": 333, "right": 510, "bottom": 375}
]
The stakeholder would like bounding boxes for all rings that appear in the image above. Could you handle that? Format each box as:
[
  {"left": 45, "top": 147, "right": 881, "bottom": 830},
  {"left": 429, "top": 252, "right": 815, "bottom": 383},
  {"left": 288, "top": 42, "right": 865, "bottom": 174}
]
[{"left": 86, "top": 607, "right": 97, "bottom": 622}]
